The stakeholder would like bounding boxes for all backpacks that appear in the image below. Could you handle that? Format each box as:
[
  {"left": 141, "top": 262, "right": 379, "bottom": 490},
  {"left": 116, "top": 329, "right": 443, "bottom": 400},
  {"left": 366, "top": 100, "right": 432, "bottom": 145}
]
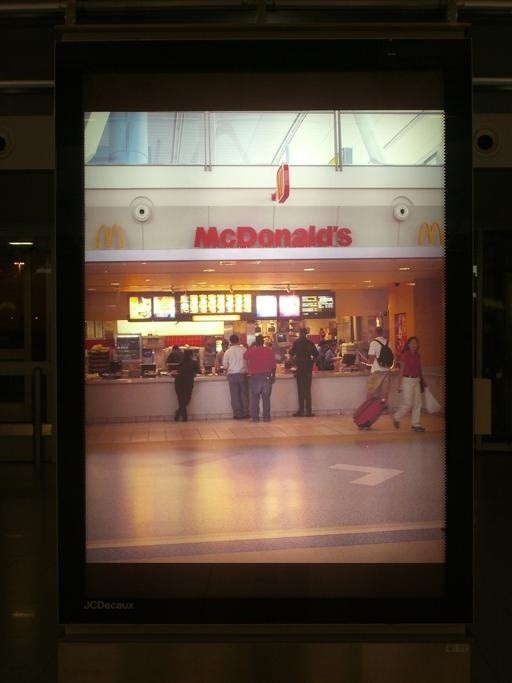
[{"left": 376, "top": 340, "right": 393, "bottom": 367}]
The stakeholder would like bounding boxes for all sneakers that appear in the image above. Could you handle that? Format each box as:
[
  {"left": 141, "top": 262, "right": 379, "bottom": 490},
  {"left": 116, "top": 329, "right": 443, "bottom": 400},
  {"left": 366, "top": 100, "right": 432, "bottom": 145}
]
[
  {"left": 183, "top": 411, "right": 187, "bottom": 421},
  {"left": 394, "top": 419, "right": 400, "bottom": 429},
  {"left": 307, "top": 412, "right": 315, "bottom": 417},
  {"left": 411, "top": 426, "right": 425, "bottom": 432},
  {"left": 175, "top": 410, "right": 180, "bottom": 420},
  {"left": 293, "top": 412, "right": 304, "bottom": 417}
]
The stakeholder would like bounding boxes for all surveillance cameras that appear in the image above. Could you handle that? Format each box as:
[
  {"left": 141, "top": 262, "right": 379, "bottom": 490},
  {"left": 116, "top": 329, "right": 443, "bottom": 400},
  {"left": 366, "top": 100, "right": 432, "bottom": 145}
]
[{"left": 395, "top": 282, "right": 400, "bottom": 287}]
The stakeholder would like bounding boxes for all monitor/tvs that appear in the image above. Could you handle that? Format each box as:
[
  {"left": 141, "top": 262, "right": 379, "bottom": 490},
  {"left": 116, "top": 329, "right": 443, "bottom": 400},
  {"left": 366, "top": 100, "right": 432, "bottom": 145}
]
[
  {"left": 276, "top": 333, "right": 288, "bottom": 344},
  {"left": 342, "top": 353, "right": 357, "bottom": 366}
]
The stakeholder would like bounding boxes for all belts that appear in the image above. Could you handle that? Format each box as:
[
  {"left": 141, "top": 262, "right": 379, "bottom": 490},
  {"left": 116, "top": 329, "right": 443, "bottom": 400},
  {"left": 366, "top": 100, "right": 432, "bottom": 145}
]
[{"left": 404, "top": 375, "right": 419, "bottom": 378}]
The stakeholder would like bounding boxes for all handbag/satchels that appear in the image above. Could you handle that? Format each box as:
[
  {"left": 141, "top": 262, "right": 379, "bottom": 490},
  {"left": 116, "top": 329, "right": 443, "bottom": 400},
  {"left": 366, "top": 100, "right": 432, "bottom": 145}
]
[{"left": 420, "top": 379, "right": 426, "bottom": 393}]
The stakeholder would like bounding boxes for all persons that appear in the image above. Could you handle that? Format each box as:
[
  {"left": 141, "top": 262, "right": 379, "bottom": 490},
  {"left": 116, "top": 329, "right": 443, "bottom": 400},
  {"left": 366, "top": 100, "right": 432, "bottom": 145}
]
[
  {"left": 366, "top": 326, "right": 395, "bottom": 401},
  {"left": 392, "top": 336, "right": 426, "bottom": 432},
  {"left": 165, "top": 327, "right": 342, "bottom": 423}
]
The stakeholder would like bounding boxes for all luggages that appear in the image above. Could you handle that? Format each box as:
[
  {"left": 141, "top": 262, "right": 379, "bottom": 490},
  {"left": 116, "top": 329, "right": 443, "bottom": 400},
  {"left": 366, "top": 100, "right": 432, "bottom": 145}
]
[{"left": 353, "top": 388, "right": 391, "bottom": 430}]
